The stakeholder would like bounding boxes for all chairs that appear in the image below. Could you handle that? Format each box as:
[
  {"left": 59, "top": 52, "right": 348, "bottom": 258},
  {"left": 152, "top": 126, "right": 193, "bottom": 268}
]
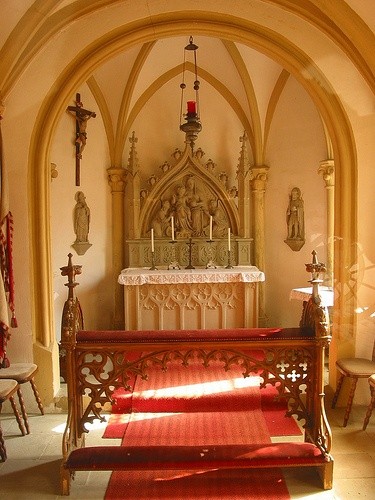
[
  {"left": 332, "top": 342, "right": 375, "bottom": 430},
  {"left": 0, "top": 360, "right": 47, "bottom": 464}
]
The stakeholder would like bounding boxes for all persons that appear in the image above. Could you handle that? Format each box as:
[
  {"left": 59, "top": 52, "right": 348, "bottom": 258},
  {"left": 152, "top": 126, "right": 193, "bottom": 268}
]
[
  {"left": 286, "top": 187, "right": 304, "bottom": 240},
  {"left": 74, "top": 192, "right": 90, "bottom": 243},
  {"left": 68, "top": 110, "right": 95, "bottom": 159},
  {"left": 146, "top": 200, "right": 175, "bottom": 238},
  {"left": 191, "top": 199, "right": 228, "bottom": 239},
  {"left": 171, "top": 177, "right": 195, "bottom": 232}
]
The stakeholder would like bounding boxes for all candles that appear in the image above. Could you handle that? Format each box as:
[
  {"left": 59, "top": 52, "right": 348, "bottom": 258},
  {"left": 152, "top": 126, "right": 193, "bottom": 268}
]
[
  {"left": 226, "top": 226, "right": 234, "bottom": 253},
  {"left": 149, "top": 226, "right": 157, "bottom": 256},
  {"left": 170, "top": 214, "right": 178, "bottom": 244},
  {"left": 207, "top": 214, "right": 215, "bottom": 242},
  {"left": 185, "top": 95, "right": 197, "bottom": 117}
]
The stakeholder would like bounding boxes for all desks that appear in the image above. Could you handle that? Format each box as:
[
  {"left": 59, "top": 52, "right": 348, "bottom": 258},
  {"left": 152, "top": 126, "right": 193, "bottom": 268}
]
[
  {"left": 289, "top": 278, "right": 334, "bottom": 332},
  {"left": 115, "top": 259, "right": 268, "bottom": 334}
]
